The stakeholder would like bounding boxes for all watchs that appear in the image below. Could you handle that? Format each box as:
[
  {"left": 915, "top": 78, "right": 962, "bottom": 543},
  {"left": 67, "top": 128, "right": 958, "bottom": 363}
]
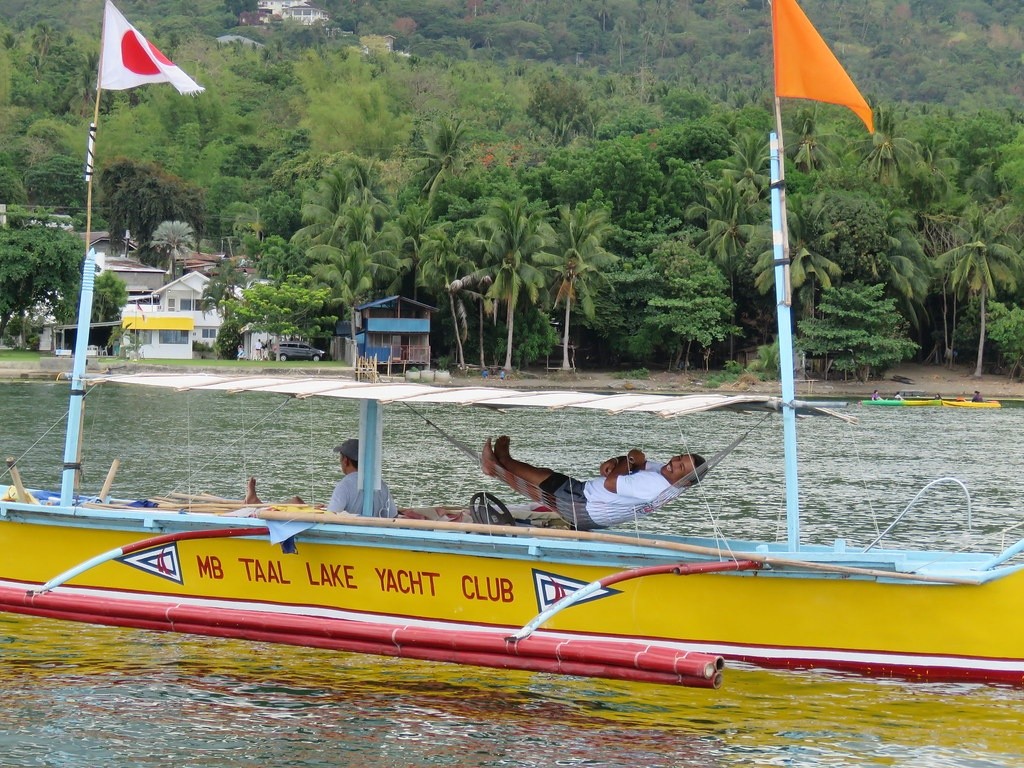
[{"left": 626, "top": 457, "right": 636, "bottom": 468}]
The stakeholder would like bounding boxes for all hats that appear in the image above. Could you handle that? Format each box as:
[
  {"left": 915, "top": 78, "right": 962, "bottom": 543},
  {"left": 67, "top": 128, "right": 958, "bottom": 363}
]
[{"left": 333, "top": 439, "right": 358, "bottom": 461}]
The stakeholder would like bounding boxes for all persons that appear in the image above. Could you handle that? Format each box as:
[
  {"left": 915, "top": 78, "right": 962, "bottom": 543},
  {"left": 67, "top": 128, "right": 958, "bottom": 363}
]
[
  {"left": 482, "top": 367, "right": 488, "bottom": 378},
  {"left": 243, "top": 439, "right": 398, "bottom": 518},
  {"left": 238, "top": 345, "right": 243, "bottom": 355},
  {"left": 491, "top": 368, "right": 495, "bottom": 374},
  {"left": 482, "top": 435, "right": 707, "bottom": 530},
  {"left": 501, "top": 369, "right": 505, "bottom": 380},
  {"left": 256, "top": 339, "right": 271, "bottom": 360}
]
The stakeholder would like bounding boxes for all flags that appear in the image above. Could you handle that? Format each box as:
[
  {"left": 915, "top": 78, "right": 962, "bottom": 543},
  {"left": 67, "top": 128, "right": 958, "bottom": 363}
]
[
  {"left": 773, "top": 0, "right": 873, "bottom": 133},
  {"left": 97, "top": 0, "right": 205, "bottom": 96}
]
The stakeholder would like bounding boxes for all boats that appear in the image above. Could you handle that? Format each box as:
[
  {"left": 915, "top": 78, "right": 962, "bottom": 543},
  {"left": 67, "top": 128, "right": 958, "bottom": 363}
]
[
  {"left": 861, "top": 399, "right": 1004, "bottom": 407},
  {"left": 0, "top": 131, "right": 1023, "bottom": 691}
]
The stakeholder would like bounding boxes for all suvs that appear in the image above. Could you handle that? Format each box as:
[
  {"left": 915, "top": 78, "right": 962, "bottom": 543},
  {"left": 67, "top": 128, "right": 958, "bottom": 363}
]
[{"left": 278, "top": 342, "right": 327, "bottom": 362}]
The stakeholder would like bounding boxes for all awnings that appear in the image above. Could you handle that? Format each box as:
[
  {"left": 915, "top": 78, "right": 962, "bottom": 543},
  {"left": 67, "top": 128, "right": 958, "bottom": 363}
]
[{"left": 122, "top": 317, "right": 194, "bottom": 330}]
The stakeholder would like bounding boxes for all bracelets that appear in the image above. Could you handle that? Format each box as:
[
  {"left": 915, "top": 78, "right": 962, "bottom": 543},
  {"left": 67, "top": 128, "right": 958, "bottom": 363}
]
[{"left": 614, "top": 457, "right": 618, "bottom": 464}]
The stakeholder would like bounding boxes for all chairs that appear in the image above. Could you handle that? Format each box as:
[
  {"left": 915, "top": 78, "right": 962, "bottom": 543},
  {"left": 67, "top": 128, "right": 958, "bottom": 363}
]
[{"left": 102, "top": 346, "right": 108, "bottom": 355}]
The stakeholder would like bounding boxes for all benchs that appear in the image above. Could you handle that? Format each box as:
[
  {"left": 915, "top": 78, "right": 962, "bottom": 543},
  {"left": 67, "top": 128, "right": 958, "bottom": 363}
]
[{"left": 543, "top": 367, "right": 576, "bottom": 374}]
